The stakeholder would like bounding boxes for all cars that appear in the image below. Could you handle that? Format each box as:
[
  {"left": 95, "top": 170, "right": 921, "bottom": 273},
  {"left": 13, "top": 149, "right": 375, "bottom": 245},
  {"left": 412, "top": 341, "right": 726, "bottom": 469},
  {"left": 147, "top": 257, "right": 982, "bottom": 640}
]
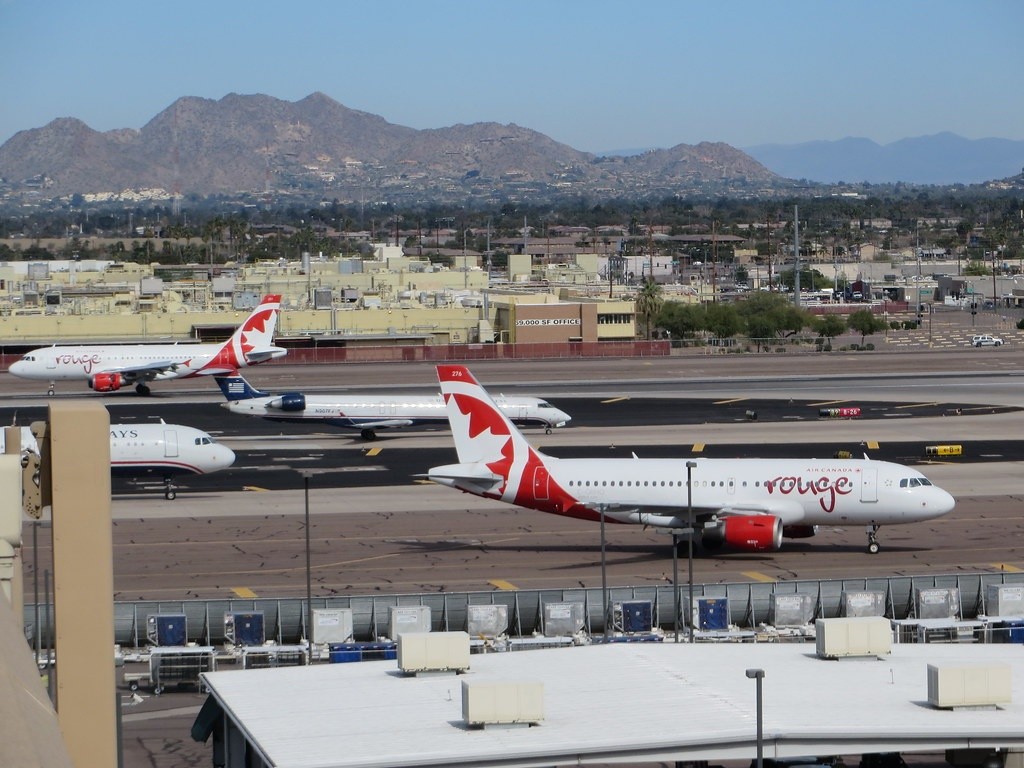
[
  {"left": 852, "top": 291, "right": 863, "bottom": 299},
  {"left": 984, "top": 301, "right": 994, "bottom": 311}
]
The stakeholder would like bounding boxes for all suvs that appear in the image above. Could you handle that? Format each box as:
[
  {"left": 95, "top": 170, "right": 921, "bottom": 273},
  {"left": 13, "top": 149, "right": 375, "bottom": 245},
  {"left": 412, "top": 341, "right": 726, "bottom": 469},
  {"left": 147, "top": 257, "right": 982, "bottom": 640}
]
[{"left": 971, "top": 336, "right": 1004, "bottom": 347}]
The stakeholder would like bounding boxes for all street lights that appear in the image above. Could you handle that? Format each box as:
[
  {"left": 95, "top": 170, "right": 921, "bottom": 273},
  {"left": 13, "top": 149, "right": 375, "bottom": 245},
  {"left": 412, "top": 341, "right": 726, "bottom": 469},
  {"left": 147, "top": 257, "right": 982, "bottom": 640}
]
[
  {"left": 302, "top": 470, "right": 314, "bottom": 645},
  {"left": 685, "top": 461, "right": 699, "bottom": 648},
  {"left": 746, "top": 668, "right": 767, "bottom": 768}
]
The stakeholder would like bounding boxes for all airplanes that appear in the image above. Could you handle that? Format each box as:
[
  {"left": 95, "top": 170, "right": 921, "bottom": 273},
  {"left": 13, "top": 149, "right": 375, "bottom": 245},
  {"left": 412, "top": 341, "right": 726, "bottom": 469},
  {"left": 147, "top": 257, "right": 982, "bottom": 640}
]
[
  {"left": 405, "top": 360, "right": 957, "bottom": 556},
  {"left": 191, "top": 363, "right": 574, "bottom": 439},
  {"left": 0, "top": 420, "right": 236, "bottom": 502},
  {"left": 7, "top": 294, "right": 290, "bottom": 395}
]
